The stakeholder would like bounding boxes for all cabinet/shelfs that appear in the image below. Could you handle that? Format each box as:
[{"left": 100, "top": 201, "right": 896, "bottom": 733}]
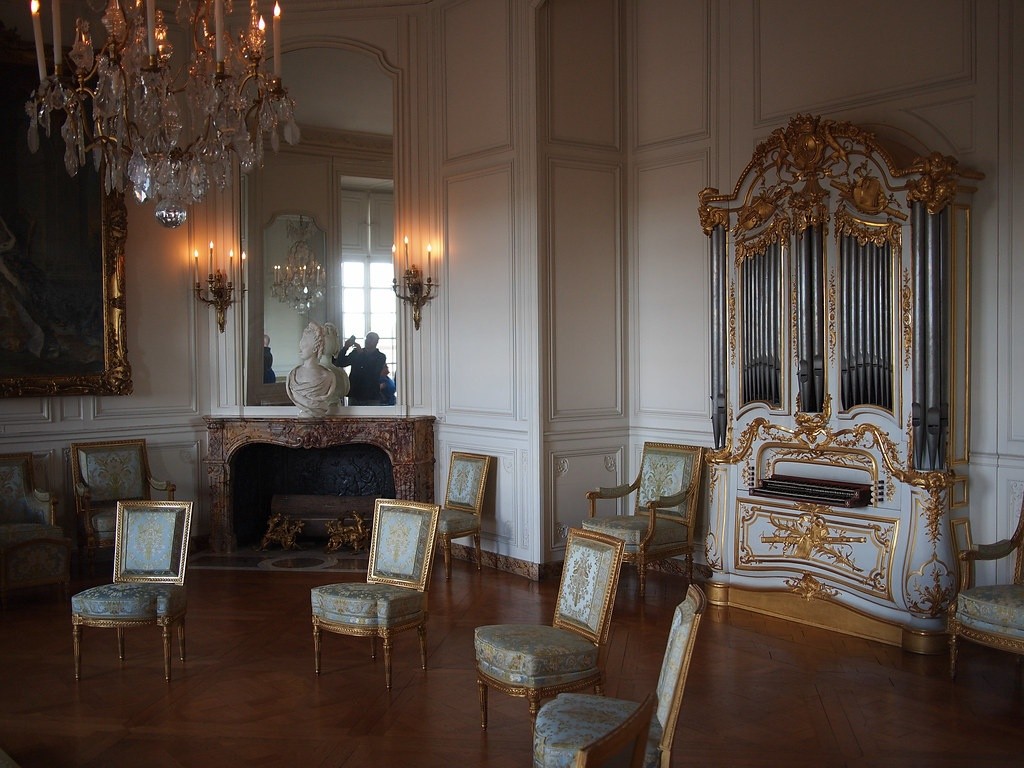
[{"left": 706, "top": 112, "right": 984, "bottom": 657}]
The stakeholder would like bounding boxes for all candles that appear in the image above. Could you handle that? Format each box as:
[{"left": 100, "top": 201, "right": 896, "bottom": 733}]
[
  {"left": 275, "top": 265, "right": 321, "bottom": 285},
  {"left": 31, "top": 0, "right": 47, "bottom": 83},
  {"left": 404, "top": 236, "right": 409, "bottom": 269},
  {"left": 194, "top": 249, "right": 199, "bottom": 283},
  {"left": 259, "top": 15, "right": 266, "bottom": 39},
  {"left": 209, "top": 240, "right": 214, "bottom": 273},
  {"left": 273, "top": 1, "right": 282, "bottom": 78},
  {"left": 228, "top": 249, "right": 233, "bottom": 282},
  {"left": 51, "top": 0, "right": 62, "bottom": 65},
  {"left": 427, "top": 244, "right": 432, "bottom": 278},
  {"left": 392, "top": 242, "right": 397, "bottom": 279},
  {"left": 214, "top": 0, "right": 224, "bottom": 62},
  {"left": 147, "top": 0, "right": 156, "bottom": 55}
]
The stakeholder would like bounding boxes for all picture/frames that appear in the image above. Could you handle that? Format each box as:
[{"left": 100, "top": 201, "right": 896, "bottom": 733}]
[{"left": 1, "top": 38, "right": 133, "bottom": 398}]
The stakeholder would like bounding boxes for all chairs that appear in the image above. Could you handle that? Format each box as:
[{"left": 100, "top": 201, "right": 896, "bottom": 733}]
[
  {"left": 582, "top": 442, "right": 704, "bottom": 596},
  {"left": 949, "top": 496, "right": 1023, "bottom": 686},
  {"left": 474, "top": 528, "right": 626, "bottom": 731},
  {"left": 70, "top": 439, "right": 177, "bottom": 586},
  {"left": 72, "top": 500, "right": 195, "bottom": 684},
  {"left": 434, "top": 450, "right": 490, "bottom": 580},
  {"left": 312, "top": 498, "right": 441, "bottom": 692},
  {"left": 0, "top": 452, "right": 72, "bottom": 611},
  {"left": 533, "top": 584, "right": 707, "bottom": 768},
  {"left": 576, "top": 690, "right": 656, "bottom": 768}
]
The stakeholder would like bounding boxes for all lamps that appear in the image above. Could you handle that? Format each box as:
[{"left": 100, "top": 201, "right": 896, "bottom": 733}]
[
  {"left": 272, "top": 213, "right": 329, "bottom": 314},
  {"left": 25, "top": 0, "right": 301, "bottom": 229}
]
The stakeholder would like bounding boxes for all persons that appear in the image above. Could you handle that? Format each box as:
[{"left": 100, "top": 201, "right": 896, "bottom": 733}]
[
  {"left": 318, "top": 322, "right": 351, "bottom": 406},
  {"left": 264, "top": 334, "right": 273, "bottom": 383},
  {"left": 285, "top": 322, "right": 336, "bottom": 416},
  {"left": 336, "top": 332, "right": 386, "bottom": 406}
]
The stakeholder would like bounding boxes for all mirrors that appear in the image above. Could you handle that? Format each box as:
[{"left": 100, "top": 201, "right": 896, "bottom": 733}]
[{"left": 240, "top": 47, "right": 398, "bottom": 406}]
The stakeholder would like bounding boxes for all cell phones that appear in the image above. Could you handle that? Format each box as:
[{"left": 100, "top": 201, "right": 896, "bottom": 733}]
[{"left": 349, "top": 335, "right": 356, "bottom": 343}]
